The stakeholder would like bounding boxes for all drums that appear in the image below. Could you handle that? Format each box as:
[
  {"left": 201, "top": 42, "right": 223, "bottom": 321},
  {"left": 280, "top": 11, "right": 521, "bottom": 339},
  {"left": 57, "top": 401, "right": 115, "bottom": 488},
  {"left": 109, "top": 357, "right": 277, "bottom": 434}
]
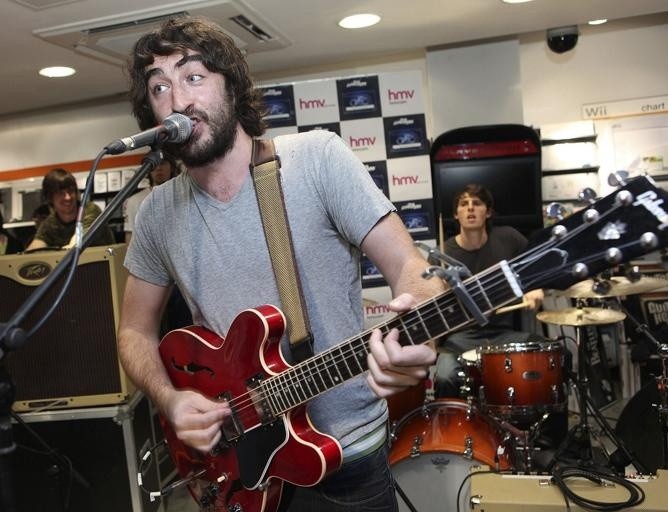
[
  {"left": 386, "top": 395, "right": 516, "bottom": 512},
  {"left": 474, "top": 340, "right": 567, "bottom": 416}
]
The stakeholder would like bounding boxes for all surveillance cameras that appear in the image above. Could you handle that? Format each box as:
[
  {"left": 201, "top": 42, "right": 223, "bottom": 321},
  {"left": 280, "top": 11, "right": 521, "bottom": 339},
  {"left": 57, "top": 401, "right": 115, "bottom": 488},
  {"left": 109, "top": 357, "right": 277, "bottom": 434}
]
[{"left": 545, "top": 26, "right": 580, "bottom": 54}]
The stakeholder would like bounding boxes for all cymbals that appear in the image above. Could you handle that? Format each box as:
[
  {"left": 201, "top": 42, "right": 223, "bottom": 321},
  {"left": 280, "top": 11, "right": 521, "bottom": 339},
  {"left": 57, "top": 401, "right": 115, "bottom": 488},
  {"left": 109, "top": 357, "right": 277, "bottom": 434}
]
[
  {"left": 535, "top": 305, "right": 627, "bottom": 327},
  {"left": 555, "top": 275, "right": 668, "bottom": 299}
]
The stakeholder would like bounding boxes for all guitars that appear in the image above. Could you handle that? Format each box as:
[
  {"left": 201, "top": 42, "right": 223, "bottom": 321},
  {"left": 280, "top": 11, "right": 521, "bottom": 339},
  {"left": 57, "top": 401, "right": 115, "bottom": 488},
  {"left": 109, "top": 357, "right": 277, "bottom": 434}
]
[{"left": 136, "top": 173, "right": 668, "bottom": 512}]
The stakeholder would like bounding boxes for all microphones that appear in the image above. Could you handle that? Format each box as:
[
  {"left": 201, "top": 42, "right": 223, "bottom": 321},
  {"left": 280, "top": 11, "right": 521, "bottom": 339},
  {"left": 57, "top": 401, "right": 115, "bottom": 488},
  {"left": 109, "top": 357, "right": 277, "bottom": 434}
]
[{"left": 104, "top": 113, "right": 194, "bottom": 157}]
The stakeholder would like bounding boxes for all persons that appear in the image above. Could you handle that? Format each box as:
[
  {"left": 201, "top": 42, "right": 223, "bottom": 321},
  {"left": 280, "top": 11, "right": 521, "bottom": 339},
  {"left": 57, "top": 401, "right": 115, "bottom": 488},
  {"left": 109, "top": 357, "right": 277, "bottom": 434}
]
[
  {"left": 118, "top": 15, "right": 445, "bottom": 512},
  {"left": 24, "top": 204, "right": 51, "bottom": 248},
  {"left": 23, "top": 169, "right": 116, "bottom": 253},
  {"left": 122, "top": 155, "right": 177, "bottom": 247},
  {"left": 1, "top": 212, "right": 24, "bottom": 255},
  {"left": 427, "top": 184, "right": 545, "bottom": 400}
]
